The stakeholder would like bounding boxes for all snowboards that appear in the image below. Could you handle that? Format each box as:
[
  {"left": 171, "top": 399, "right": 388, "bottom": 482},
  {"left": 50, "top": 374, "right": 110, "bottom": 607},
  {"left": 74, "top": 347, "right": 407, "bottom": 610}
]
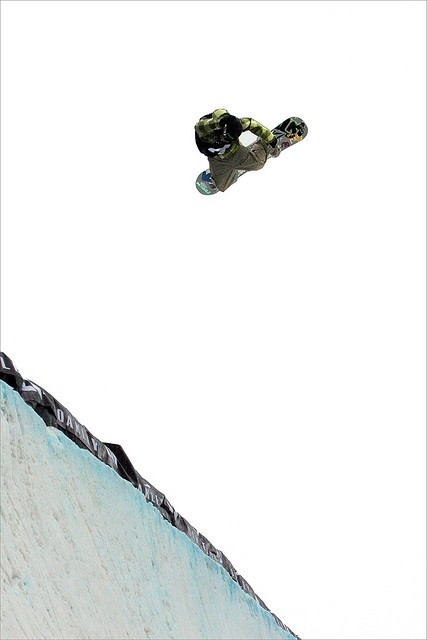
[{"left": 195, "top": 116, "right": 308, "bottom": 195}]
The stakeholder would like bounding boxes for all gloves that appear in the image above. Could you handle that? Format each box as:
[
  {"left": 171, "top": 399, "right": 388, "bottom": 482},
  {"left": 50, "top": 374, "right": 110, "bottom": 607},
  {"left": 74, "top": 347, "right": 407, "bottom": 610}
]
[
  {"left": 270, "top": 137, "right": 278, "bottom": 148},
  {"left": 219, "top": 115, "right": 234, "bottom": 127}
]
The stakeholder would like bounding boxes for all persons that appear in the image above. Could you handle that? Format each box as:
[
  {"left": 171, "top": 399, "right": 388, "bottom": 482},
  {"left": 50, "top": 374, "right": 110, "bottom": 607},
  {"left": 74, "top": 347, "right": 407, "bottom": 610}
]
[{"left": 193, "top": 109, "right": 281, "bottom": 192}]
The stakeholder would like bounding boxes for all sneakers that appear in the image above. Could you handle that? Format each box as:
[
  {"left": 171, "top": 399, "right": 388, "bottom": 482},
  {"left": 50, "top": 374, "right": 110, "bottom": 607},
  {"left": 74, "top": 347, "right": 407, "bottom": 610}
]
[{"left": 261, "top": 138, "right": 280, "bottom": 157}]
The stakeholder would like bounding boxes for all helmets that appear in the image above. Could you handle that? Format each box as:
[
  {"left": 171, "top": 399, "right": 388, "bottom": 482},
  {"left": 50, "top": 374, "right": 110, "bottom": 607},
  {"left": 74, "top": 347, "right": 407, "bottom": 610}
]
[{"left": 216, "top": 114, "right": 243, "bottom": 143}]
